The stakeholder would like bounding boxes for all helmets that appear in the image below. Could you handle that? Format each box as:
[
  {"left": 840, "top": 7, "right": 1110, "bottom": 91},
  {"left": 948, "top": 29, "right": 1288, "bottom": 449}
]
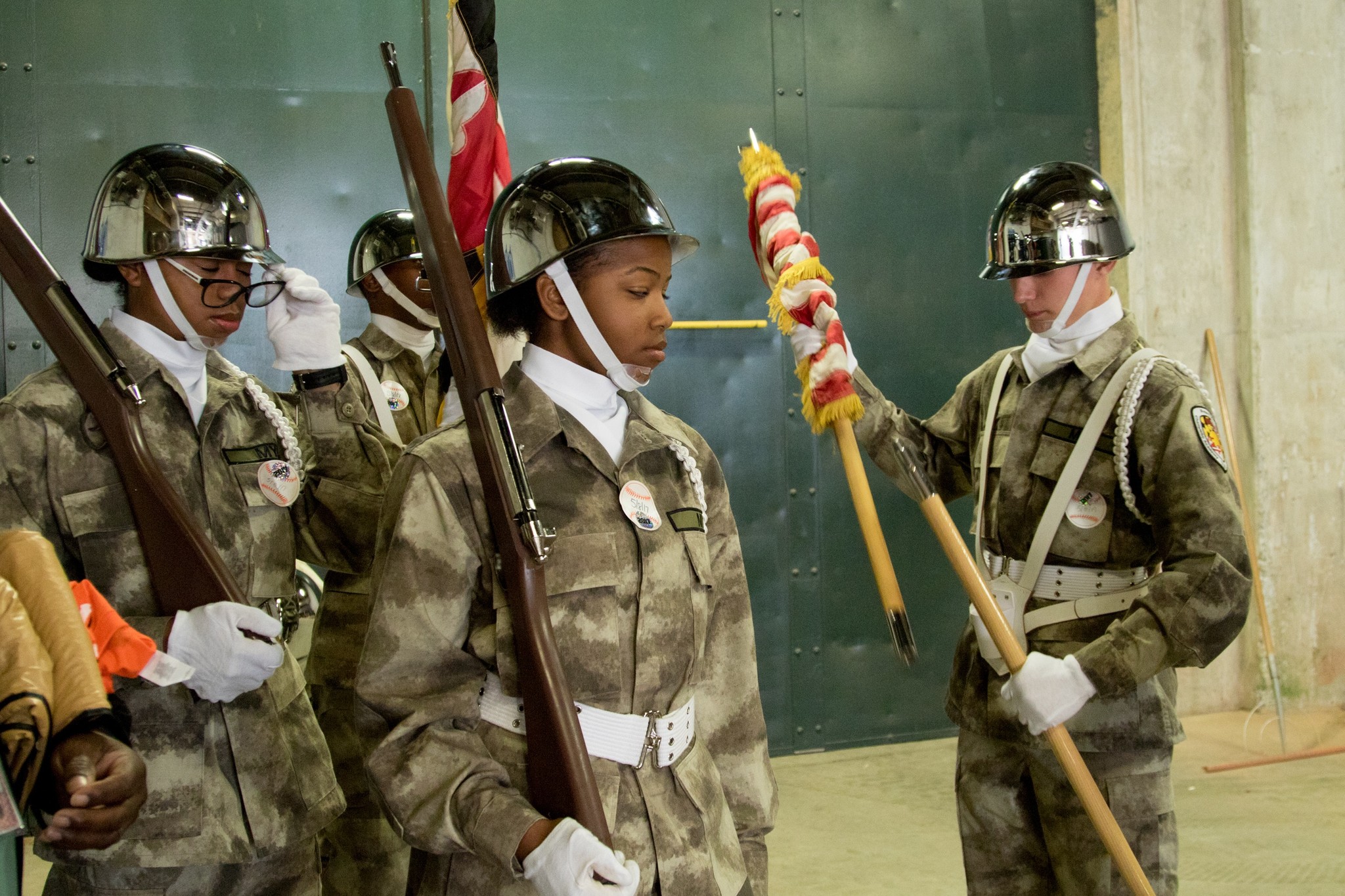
[
  {"left": 981, "top": 161, "right": 1135, "bottom": 282},
  {"left": 346, "top": 206, "right": 421, "bottom": 297},
  {"left": 482, "top": 155, "right": 699, "bottom": 299},
  {"left": 81, "top": 142, "right": 286, "bottom": 265}
]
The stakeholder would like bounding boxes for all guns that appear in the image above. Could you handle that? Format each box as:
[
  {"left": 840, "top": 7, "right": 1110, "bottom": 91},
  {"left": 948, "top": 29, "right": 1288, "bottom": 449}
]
[
  {"left": 379, "top": 41, "right": 618, "bottom": 892},
  {"left": 0, "top": 199, "right": 279, "bottom": 669}
]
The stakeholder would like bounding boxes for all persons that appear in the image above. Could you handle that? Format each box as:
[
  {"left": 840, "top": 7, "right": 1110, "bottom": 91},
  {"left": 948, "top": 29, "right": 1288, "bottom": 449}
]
[
  {"left": 843, "top": 160, "right": 1252, "bottom": 896},
  {"left": 0, "top": 133, "right": 779, "bottom": 896}
]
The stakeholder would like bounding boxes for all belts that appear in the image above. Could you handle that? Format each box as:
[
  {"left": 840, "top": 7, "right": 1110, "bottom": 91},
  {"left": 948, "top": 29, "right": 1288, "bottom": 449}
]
[
  {"left": 476, "top": 683, "right": 697, "bottom": 766},
  {"left": 979, "top": 550, "right": 1146, "bottom": 600}
]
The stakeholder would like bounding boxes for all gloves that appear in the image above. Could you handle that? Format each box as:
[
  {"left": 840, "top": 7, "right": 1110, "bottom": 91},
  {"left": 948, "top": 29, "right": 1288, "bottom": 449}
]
[
  {"left": 521, "top": 816, "right": 639, "bottom": 895},
  {"left": 1000, "top": 651, "right": 1099, "bottom": 734},
  {"left": 791, "top": 323, "right": 859, "bottom": 387},
  {"left": 259, "top": 265, "right": 348, "bottom": 372},
  {"left": 166, "top": 598, "right": 286, "bottom": 704}
]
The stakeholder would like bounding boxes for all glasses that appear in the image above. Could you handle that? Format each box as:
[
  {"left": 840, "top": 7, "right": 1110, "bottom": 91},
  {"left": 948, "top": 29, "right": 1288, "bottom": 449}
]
[{"left": 165, "top": 257, "right": 287, "bottom": 308}]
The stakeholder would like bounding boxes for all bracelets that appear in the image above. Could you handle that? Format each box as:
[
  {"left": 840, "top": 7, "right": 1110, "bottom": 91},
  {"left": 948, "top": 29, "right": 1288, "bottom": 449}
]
[{"left": 291, "top": 364, "right": 348, "bottom": 392}]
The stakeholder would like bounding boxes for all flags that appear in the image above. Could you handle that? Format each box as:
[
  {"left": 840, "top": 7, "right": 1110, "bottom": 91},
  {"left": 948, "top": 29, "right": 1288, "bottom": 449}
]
[
  {"left": 737, "top": 141, "right": 864, "bottom": 436},
  {"left": 435, "top": 0, "right": 515, "bottom": 428}
]
